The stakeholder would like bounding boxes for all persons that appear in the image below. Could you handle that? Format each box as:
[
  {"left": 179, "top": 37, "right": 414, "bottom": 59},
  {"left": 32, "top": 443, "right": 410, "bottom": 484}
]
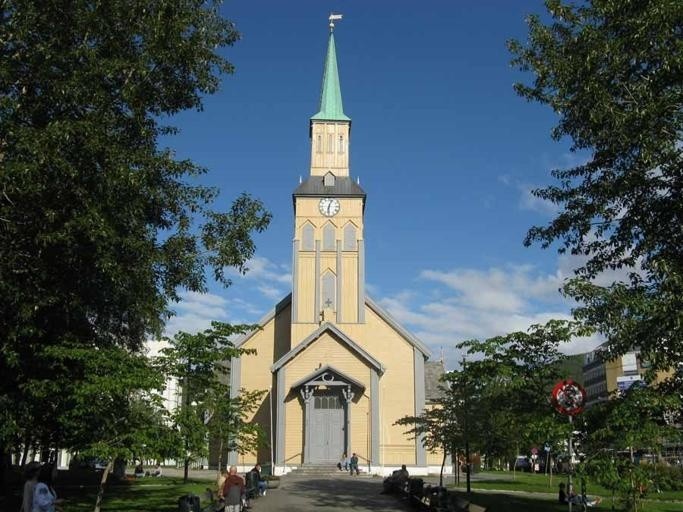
[
  {"left": 559, "top": 483, "right": 569, "bottom": 504},
  {"left": 32, "top": 462, "right": 57, "bottom": 512},
  {"left": 218, "top": 463, "right": 266, "bottom": 512},
  {"left": 340, "top": 455, "right": 347, "bottom": 471},
  {"left": 135, "top": 463, "right": 145, "bottom": 478},
  {"left": 152, "top": 464, "right": 162, "bottom": 478},
  {"left": 20, "top": 462, "right": 41, "bottom": 512},
  {"left": 350, "top": 454, "right": 359, "bottom": 476},
  {"left": 380, "top": 465, "right": 408, "bottom": 495}
]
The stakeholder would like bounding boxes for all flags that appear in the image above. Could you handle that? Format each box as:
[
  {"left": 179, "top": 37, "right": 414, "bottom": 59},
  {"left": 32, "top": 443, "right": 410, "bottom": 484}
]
[{"left": 329, "top": 14, "right": 342, "bottom": 19}]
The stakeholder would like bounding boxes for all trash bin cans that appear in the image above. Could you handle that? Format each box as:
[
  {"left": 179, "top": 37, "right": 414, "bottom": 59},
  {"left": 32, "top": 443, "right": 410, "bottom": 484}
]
[{"left": 260, "top": 462, "right": 271, "bottom": 475}]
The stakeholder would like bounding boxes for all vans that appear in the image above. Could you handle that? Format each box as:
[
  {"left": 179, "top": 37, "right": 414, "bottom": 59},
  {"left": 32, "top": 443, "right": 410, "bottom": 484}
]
[{"left": 515, "top": 456, "right": 544, "bottom": 473}]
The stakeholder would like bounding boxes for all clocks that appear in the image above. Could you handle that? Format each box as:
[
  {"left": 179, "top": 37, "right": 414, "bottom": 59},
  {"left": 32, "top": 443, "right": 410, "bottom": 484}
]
[{"left": 317, "top": 196, "right": 340, "bottom": 217}]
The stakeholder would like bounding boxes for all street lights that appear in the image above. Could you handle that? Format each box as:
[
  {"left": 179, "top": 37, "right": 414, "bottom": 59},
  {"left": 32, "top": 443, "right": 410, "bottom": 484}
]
[{"left": 459, "top": 340, "right": 470, "bottom": 493}]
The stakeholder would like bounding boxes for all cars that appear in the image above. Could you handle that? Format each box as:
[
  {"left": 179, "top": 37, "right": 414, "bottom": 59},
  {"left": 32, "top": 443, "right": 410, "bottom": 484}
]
[{"left": 71, "top": 453, "right": 112, "bottom": 475}]
